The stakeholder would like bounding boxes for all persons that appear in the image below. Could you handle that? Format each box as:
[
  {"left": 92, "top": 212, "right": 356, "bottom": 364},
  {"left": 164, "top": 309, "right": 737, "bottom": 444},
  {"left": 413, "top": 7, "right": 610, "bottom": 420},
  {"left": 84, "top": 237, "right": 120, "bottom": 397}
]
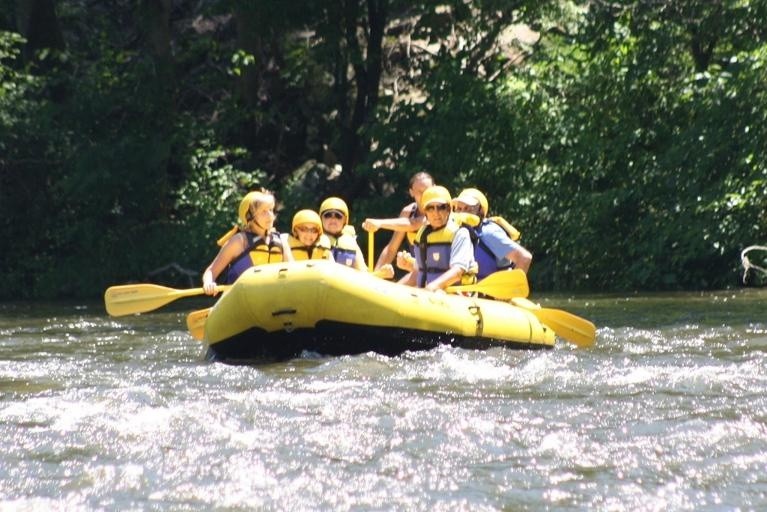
[
  {"left": 396, "top": 185, "right": 478, "bottom": 298},
  {"left": 260, "top": 188, "right": 335, "bottom": 263},
  {"left": 200, "top": 189, "right": 293, "bottom": 297},
  {"left": 448, "top": 187, "right": 533, "bottom": 303},
  {"left": 361, "top": 170, "right": 438, "bottom": 287},
  {"left": 317, "top": 195, "right": 396, "bottom": 280}
]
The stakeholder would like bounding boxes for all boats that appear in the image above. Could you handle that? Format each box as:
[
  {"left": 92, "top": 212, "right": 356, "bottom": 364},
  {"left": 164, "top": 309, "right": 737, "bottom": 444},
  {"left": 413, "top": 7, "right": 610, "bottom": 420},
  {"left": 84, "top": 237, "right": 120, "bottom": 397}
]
[{"left": 203, "top": 259, "right": 555, "bottom": 359}]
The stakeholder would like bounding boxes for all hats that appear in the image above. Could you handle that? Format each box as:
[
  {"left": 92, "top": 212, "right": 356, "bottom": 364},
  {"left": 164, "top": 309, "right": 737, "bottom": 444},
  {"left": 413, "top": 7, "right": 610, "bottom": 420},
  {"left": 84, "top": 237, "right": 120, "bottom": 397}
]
[{"left": 453, "top": 193, "right": 479, "bottom": 207}]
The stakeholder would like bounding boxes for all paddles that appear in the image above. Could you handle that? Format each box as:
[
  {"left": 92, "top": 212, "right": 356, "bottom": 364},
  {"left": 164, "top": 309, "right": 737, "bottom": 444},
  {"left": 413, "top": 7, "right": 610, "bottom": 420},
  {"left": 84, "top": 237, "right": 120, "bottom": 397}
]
[
  {"left": 509, "top": 301, "right": 596, "bottom": 348},
  {"left": 187, "top": 307, "right": 216, "bottom": 339},
  {"left": 445, "top": 269, "right": 529, "bottom": 299},
  {"left": 104, "top": 271, "right": 387, "bottom": 316}
]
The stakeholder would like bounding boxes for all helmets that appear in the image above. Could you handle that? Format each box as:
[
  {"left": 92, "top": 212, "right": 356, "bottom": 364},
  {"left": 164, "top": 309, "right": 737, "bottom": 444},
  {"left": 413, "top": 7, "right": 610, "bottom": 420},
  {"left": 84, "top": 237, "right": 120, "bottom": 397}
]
[
  {"left": 318, "top": 197, "right": 350, "bottom": 225},
  {"left": 459, "top": 187, "right": 489, "bottom": 218},
  {"left": 239, "top": 191, "right": 263, "bottom": 225},
  {"left": 291, "top": 209, "right": 323, "bottom": 236},
  {"left": 419, "top": 184, "right": 452, "bottom": 210}
]
